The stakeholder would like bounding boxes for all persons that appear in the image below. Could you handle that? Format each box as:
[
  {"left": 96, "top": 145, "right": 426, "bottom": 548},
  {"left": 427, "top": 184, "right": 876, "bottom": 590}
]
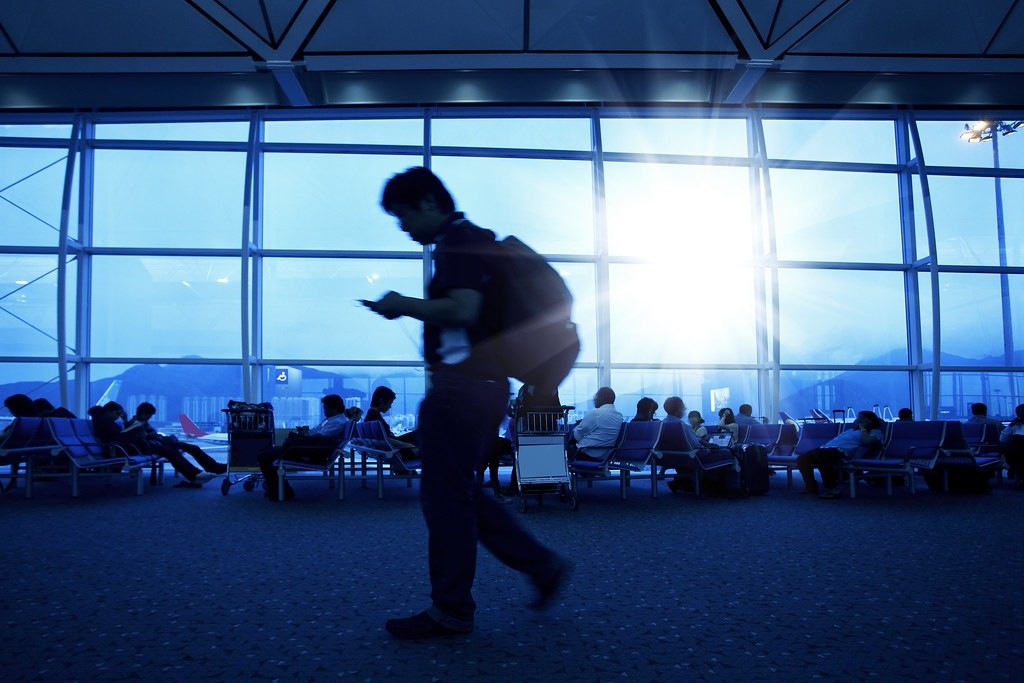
[
  {"left": 896, "top": 408, "right": 913, "bottom": 421},
  {"left": 364, "top": 386, "right": 419, "bottom": 460},
  {"left": 257, "top": 395, "right": 348, "bottom": 498},
  {"left": 346, "top": 407, "right": 363, "bottom": 422},
  {"left": 567, "top": 387, "right": 624, "bottom": 462},
  {"left": 797, "top": 411, "right": 884, "bottom": 499},
  {"left": 475, "top": 386, "right": 563, "bottom": 496},
  {"left": 965, "top": 403, "right": 1024, "bottom": 479},
  {"left": 735, "top": 404, "right": 776, "bottom": 476},
  {"left": 0, "top": 394, "right": 53, "bottom": 490},
  {"left": 369, "top": 167, "right": 567, "bottom": 641},
  {"left": 631, "top": 397, "right": 738, "bottom": 492},
  {"left": 88, "top": 401, "right": 228, "bottom": 485}
]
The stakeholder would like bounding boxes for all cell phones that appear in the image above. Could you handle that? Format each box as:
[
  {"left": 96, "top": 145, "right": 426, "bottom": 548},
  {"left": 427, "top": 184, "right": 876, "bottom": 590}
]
[{"left": 356, "top": 299, "right": 375, "bottom": 303}]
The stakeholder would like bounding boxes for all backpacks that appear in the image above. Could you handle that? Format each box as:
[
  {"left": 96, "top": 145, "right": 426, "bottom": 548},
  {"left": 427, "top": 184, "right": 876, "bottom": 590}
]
[{"left": 436, "top": 235, "right": 581, "bottom": 395}]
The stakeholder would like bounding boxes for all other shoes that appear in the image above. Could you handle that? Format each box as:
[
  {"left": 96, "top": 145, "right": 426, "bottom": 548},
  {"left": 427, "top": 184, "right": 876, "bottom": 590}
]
[
  {"left": 262, "top": 480, "right": 280, "bottom": 498},
  {"left": 205, "top": 463, "right": 227, "bottom": 475},
  {"left": 184, "top": 467, "right": 215, "bottom": 482},
  {"left": 530, "top": 552, "right": 578, "bottom": 612},
  {"left": 505, "top": 482, "right": 519, "bottom": 496},
  {"left": 667, "top": 480, "right": 680, "bottom": 493},
  {"left": 483, "top": 479, "right": 502, "bottom": 493},
  {"left": 385, "top": 610, "right": 474, "bottom": 641},
  {"left": 539, "top": 482, "right": 558, "bottom": 493},
  {"left": 684, "top": 483, "right": 695, "bottom": 491},
  {"left": 798, "top": 485, "right": 820, "bottom": 495},
  {"left": 831, "top": 481, "right": 842, "bottom": 494}
]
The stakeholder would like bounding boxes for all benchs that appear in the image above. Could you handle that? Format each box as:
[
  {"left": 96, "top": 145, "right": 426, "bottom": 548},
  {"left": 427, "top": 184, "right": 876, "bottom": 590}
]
[{"left": 0, "top": 418, "right": 1003, "bottom": 501}]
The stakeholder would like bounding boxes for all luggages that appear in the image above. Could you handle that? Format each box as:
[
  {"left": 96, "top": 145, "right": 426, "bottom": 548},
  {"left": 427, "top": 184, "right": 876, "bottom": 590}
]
[{"left": 730, "top": 442, "right": 770, "bottom": 497}]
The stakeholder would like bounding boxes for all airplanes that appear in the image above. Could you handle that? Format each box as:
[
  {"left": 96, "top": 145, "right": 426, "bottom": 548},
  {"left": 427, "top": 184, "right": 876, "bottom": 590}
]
[
  {"left": 1, "top": 379, "right": 171, "bottom": 430},
  {"left": 176, "top": 415, "right": 227, "bottom": 454},
  {"left": 779, "top": 410, "right": 833, "bottom": 429}
]
